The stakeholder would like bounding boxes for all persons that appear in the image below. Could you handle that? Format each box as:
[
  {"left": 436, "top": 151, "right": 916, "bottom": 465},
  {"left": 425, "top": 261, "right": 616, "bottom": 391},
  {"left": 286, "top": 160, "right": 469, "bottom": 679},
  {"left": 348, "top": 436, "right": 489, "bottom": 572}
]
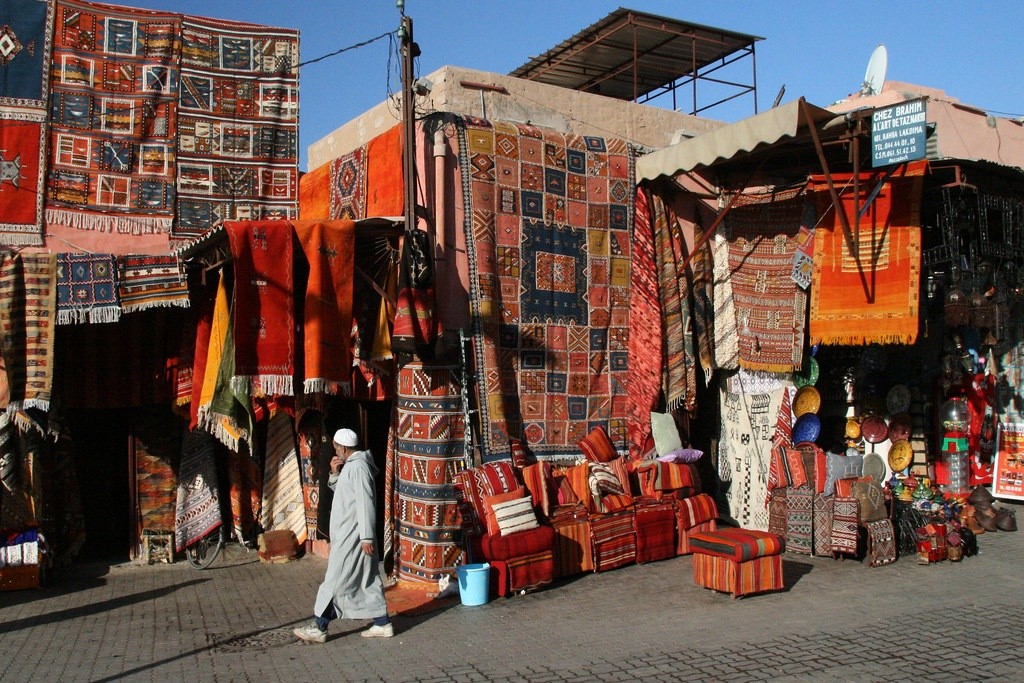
[{"left": 294, "top": 428, "right": 394, "bottom": 643}]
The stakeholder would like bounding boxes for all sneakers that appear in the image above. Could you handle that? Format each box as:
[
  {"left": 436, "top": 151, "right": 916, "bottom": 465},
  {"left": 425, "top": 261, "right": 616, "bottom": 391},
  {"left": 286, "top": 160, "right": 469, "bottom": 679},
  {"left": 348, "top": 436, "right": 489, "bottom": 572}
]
[
  {"left": 361, "top": 622, "right": 394, "bottom": 638},
  {"left": 294, "top": 621, "right": 326, "bottom": 642}
]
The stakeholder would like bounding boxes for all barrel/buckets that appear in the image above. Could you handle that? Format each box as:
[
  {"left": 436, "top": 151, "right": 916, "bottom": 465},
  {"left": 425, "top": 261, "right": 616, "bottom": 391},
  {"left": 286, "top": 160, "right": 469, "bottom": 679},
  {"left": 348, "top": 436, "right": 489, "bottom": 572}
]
[{"left": 455, "top": 562, "right": 490, "bottom": 606}]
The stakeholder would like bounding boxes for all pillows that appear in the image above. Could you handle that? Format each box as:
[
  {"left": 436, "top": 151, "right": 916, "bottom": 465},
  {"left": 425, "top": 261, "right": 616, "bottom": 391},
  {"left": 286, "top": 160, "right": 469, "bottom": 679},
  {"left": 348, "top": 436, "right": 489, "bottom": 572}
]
[
  {"left": 559, "top": 461, "right": 593, "bottom": 511},
  {"left": 609, "top": 455, "right": 633, "bottom": 497},
  {"left": 491, "top": 495, "right": 540, "bottom": 538},
  {"left": 484, "top": 486, "right": 526, "bottom": 536},
  {"left": 577, "top": 424, "right": 619, "bottom": 462},
  {"left": 546, "top": 475, "right": 583, "bottom": 507},
  {"left": 657, "top": 448, "right": 704, "bottom": 464},
  {"left": 519, "top": 458, "right": 549, "bottom": 517},
  {"left": 773, "top": 444, "right": 888, "bottom": 523}
]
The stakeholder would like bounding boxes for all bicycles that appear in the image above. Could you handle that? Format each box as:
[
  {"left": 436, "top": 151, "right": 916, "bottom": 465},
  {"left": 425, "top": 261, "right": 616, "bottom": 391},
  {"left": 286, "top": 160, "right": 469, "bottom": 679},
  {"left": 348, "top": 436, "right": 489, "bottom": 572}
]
[{"left": 184, "top": 522, "right": 225, "bottom": 571}]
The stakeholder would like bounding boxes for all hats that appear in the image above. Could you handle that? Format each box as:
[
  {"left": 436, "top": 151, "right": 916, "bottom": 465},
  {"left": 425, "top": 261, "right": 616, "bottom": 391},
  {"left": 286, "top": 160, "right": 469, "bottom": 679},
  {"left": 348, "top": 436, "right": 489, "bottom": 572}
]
[{"left": 334, "top": 428, "right": 359, "bottom": 447}]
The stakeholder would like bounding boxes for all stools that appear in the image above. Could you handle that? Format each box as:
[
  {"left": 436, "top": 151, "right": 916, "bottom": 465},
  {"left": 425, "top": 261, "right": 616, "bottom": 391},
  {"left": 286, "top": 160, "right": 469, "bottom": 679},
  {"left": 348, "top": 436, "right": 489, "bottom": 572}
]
[
  {"left": 689, "top": 527, "right": 786, "bottom": 599},
  {"left": 474, "top": 494, "right": 718, "bottom": 598}
]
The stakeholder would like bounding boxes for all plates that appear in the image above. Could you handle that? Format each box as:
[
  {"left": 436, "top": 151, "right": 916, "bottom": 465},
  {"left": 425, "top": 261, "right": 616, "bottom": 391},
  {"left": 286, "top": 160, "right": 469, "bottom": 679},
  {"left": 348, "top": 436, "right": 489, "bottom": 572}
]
[
  {"left": 888, "top": 412, "right": 913, "bottom": 444},
  {"left": 792, "top": 385, "right": 821, "bottom": 419},
  {"left": 887, "top": 384, "right": 911, "bottom": 416},
  {"left": 792, "top": 355, "right": 819, "bottom": 389},
  {"left": 792, "top": 413, "right": 821, "bottom": 444},
  {"left": 888, "top": 439, "right": 914, "bottom": 471},
  {"left": 861, "top": 453, "right": 886, "bottom": 481},
  {"left": 861, "top": 415, "right": 887, "bottom": 444}
]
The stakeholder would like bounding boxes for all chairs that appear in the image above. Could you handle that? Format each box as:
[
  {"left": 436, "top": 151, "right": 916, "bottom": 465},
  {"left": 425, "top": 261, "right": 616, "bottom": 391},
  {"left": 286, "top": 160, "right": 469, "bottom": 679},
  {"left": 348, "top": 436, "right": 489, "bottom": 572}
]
[{"left": 834, "top": 476, "right": 897, "bottom": 566}]
